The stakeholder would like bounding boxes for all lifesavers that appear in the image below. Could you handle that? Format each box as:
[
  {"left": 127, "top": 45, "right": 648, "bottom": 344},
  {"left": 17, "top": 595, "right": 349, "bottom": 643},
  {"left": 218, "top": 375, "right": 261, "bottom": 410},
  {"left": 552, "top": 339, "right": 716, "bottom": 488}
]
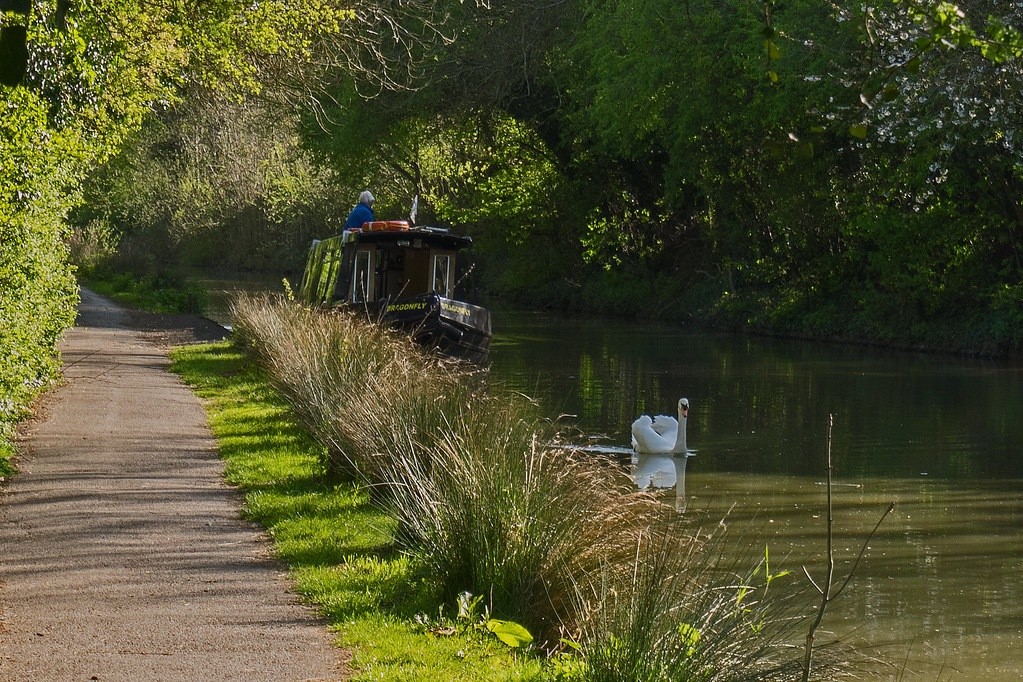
[{"left": 362, "top": 221, "right": 409, "bottom": 232}]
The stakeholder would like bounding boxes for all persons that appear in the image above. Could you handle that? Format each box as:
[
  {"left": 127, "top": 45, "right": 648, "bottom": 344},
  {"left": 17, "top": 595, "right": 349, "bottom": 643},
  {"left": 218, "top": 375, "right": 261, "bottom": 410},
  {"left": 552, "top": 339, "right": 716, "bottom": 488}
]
[{"left": 341, "top": 191, "right": 375, "bottom": 233}]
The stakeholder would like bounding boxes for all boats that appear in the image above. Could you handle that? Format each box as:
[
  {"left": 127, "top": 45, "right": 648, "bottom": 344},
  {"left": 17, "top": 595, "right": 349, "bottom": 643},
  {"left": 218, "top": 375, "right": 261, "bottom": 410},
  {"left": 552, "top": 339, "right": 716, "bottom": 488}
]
[{"left": 298, "top": 219, "right": 494, "bottom": 367}]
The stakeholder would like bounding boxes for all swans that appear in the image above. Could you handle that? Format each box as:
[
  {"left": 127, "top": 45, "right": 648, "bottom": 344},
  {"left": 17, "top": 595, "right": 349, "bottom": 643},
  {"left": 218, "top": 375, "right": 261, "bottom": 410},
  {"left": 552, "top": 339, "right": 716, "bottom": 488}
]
[{"left": 628, "top": 396, "right": 692, "bottom": 456}]
